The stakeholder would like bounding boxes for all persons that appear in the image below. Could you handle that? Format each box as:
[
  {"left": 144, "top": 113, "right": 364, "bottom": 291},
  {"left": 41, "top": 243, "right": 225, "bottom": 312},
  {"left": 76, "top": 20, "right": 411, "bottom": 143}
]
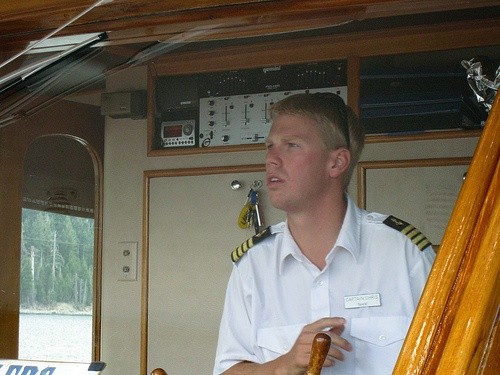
[{"left": 213, "top": 93, "right": 436, "bottom": 375}]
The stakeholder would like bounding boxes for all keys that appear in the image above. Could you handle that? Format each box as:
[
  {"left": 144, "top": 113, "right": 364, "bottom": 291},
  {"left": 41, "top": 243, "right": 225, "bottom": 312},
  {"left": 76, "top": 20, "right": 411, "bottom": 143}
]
[{"left": 238, "top": 189, "right": 265, "bottom": 235}]
